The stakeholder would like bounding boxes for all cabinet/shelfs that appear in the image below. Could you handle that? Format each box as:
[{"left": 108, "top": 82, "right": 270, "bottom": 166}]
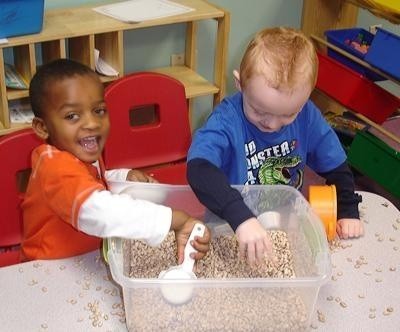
[
  {"left": 301, "top": 0, "right": 400, "bottom": 145},
  {"left": 0, "top": 0, "right": 230, "bottom": 136}
]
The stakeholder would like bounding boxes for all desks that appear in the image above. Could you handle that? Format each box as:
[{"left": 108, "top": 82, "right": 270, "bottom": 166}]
[{"left": 0, "top": 191, "right": 400, "bottom": 332}]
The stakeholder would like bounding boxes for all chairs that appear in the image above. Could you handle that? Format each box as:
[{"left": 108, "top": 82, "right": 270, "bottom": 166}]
[
  {"left": 102, "top": 72, "right": 205, "bottom": 219},
  {"left": 0, "top": 128, "right": 45, "bottom": 266}
]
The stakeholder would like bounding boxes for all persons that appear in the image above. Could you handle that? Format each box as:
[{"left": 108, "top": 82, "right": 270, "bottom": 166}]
[
  {"left": 20, "top": 58, "right": 211, "bottom": 265},
  {"left": 185, "top": 25, "right": 364, "bottom": 266}
]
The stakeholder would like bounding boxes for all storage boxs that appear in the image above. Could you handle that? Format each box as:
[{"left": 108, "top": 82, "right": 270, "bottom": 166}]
[
  {"left": 323, "top": 27, "right": 389, "bottom": 82},
  {"left": 316, "top": 51, "right": 400, "bottom": 125},
  {"left": 347, "top": 129, "right": 400, "bottom": 199},
  {"left": 103, "top": 185, "right": 331, "bottom": 332},
  {"left": 364, "top": 27, "right": 400, "bottom": 79}
]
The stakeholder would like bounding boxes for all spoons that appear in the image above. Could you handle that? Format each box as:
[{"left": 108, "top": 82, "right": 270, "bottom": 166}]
[{"left": 159, "top": 224, "right": 205, "bottom": 302}]
